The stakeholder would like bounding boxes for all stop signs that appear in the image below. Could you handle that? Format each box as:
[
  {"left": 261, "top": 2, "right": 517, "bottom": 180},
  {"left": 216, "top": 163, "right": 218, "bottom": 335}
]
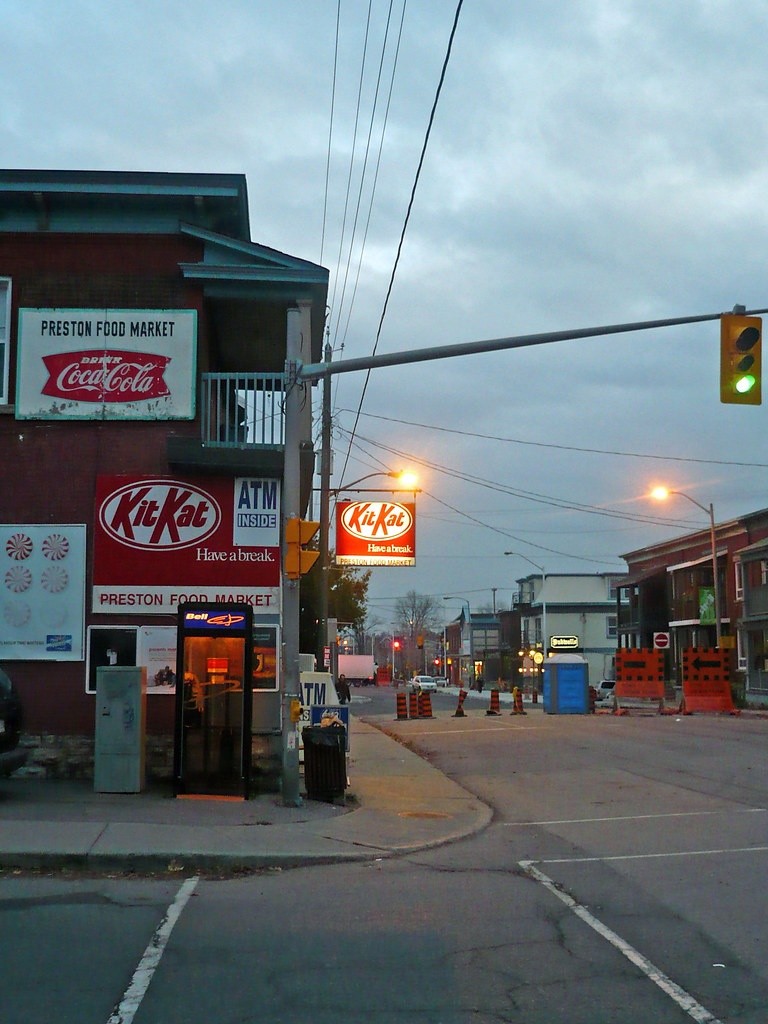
[{"left": 655, "top": 633, "right": 669, "bottom": 647}]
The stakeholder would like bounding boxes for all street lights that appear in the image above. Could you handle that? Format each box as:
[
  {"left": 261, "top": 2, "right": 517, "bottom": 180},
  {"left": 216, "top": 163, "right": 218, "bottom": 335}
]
[
  {"left": 388, "top": 619, "right": 412, "bottom": 674},
  {"left": 491, "top": 587, "right": 500, "bottom": 617},
  {"left": 654, "top": 487, "right": 722, "bottom": 647},
  {"left": 317, "top": 469, "right": 422, "bottom": 670},
  {"left": 442, "top": 595, "right": 474, "bottom": 664},
  {"left": 504, "top": 550, "right": 548, "bottom": 662}
]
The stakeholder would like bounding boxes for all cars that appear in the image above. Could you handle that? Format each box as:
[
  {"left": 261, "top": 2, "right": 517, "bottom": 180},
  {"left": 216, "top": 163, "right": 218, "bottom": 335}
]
[
  {"left": 435, "top": 676, "right": 447, "bottom": 687},
  {"left": 412, "top": 675, "right": 438, "bottom": 693}
]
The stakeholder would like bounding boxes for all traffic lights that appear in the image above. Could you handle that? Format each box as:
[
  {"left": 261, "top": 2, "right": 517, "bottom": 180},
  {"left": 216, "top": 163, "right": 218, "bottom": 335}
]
[
  {"left": 719, "top": 311, "right": 761, "bottom": 405},
  {"left": 286, "top": 514, "right": 323, "bottom": 584}
]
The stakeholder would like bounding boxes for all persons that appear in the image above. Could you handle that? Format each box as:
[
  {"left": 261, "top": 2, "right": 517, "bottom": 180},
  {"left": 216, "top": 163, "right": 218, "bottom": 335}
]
[
  {"left": 335, "top": 673, "right": 351, "bottom": 705},
  {"left": 476, "top": 674, "right": 485, "bottom": 693}
]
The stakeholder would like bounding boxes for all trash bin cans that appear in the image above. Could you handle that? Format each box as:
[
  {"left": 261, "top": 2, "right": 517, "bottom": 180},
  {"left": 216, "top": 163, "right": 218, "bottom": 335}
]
[{"left": 301, "top": 725, "right": 348, "bottom": 800}]
